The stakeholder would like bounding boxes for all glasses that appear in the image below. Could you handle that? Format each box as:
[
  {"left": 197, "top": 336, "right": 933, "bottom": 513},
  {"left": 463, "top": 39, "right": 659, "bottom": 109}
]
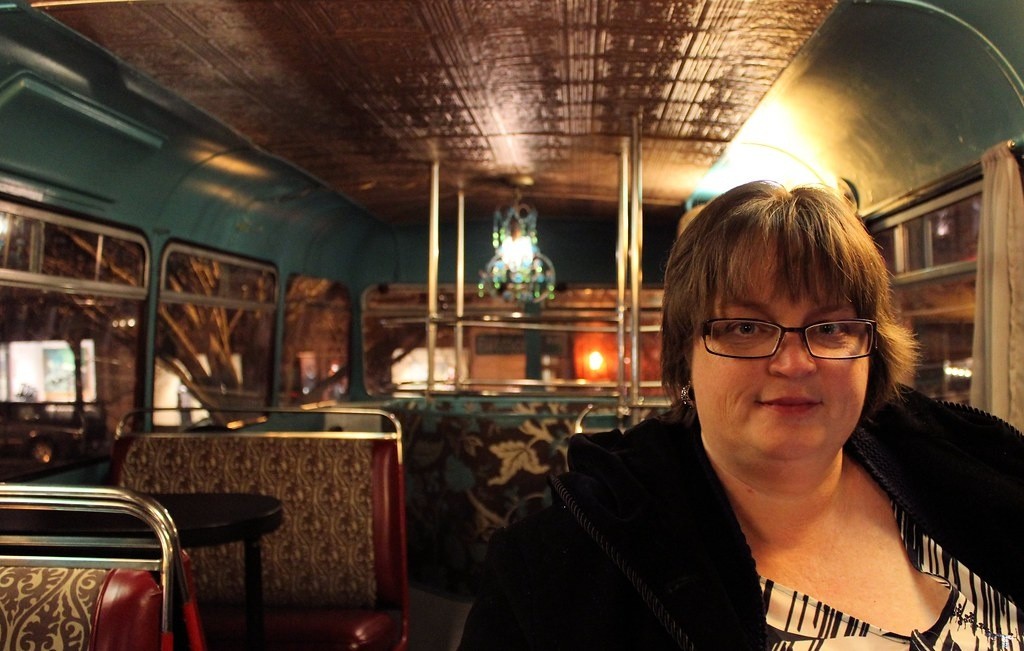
[{"left": 686, "top": 318, "right": 878, "bottom": 360}]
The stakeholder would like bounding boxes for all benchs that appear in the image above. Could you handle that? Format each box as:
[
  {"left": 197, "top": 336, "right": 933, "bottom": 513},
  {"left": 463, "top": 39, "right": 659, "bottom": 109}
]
[
  {"left": 109, "top": 397, "right": 675, "bottom": 651},
  {"left": 0, "top": 483, "right": 207, "bottom": 651}
]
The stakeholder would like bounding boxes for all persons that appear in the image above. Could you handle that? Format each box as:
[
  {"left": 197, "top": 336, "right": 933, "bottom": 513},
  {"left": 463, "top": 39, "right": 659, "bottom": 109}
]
[{"left": 458, "top": 181, "right": 1024, "bottom": 651}]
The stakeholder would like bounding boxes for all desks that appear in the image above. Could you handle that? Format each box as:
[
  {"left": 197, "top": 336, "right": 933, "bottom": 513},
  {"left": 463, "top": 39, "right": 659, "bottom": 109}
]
[{"left": 0, "top": 494, "right": 282, "bottom": 651}]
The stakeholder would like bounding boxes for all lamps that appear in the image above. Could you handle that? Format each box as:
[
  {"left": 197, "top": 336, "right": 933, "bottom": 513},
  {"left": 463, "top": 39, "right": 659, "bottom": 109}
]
[{"left": 477, "top": 174, "right": 556, "bottom": 303}]
